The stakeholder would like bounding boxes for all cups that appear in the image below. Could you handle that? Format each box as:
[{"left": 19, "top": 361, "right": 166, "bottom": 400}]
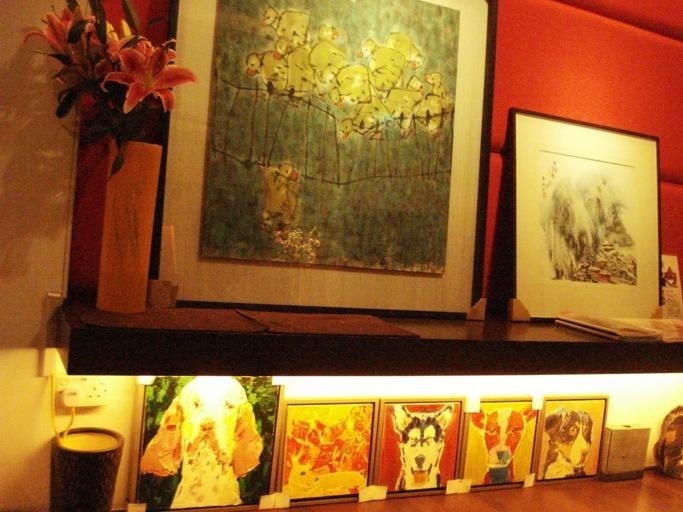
[{"left": 45, "top": 429, "right": 126, "bottom": 511}]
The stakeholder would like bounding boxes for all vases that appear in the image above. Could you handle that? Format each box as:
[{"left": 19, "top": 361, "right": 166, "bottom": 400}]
[{"left": 72, "top": 136, "right": 165, "bottom": 314}]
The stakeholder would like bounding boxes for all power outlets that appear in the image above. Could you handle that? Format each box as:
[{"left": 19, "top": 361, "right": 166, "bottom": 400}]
[{"left": 63, "top": 382, "right": 108, "bottom": 409}]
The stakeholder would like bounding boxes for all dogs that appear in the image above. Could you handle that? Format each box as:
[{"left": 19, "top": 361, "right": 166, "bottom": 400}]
[
  {"left": 140, "top": 374, "right": 265, "bottom": 509},
  {"left": 542, "top": 406, "right": 593, "bottom": 481},
  {"left": 390, "top": 403, "right": 453, "bottom": 492}
]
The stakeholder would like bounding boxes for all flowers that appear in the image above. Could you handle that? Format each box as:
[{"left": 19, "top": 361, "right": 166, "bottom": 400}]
[{"left": 22, "top": 2, "right": 198, "bottom": 174}]
